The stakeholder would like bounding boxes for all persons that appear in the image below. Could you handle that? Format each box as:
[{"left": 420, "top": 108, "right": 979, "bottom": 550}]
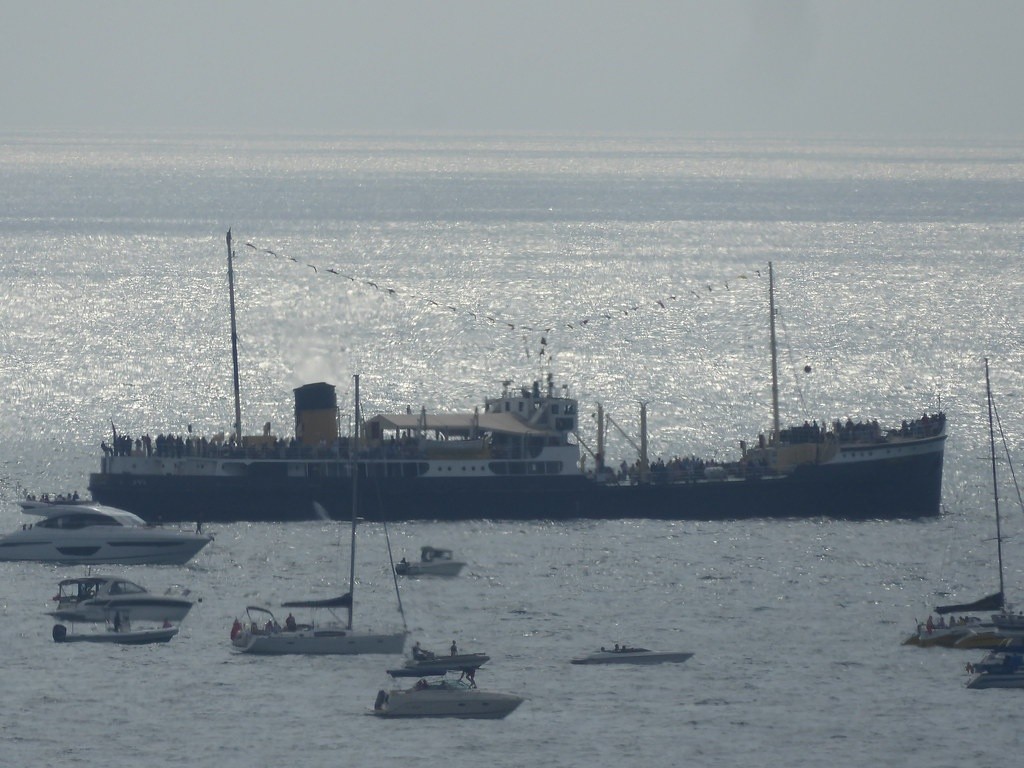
[
  {"left": 388, "top": 430, "right": 407, "bottom": 453},
  {"left": 413, "top": 641, "right": 425, "bottom": 658},
  {"left": 276, "top": 434, "right": 348, "bottom": 450},
  {"left": 457, "top": 666, "right": 477, "bottom": 689},
  {"left": 902, "top": 411, "right": 944, "bottom": 434},
  {"left": 23, "top": 491, "right": 80, "bottom": 503},
  {"left": 739, "top": 440, "right": 746, "bottom": 457},
  {"left": 286, "top": 612, "right": 296, "bottom": 631},
  {"left": 195, "top": 519, "right": 202, "bottom": 534},
  {"left": 100, "top": 432, "right": 210, "bottom": 457},
  {"left": 926, "top": 613, "right": 970, "bottom": 633},
  {"left": 451, "top": 639, "right": 458, "bottom": 657},
  {"left": 617, "top": 454, "right": 727, "bottom": 480},
  {"left": 803, "top": 415, "right": 880, "bottom": 434}
]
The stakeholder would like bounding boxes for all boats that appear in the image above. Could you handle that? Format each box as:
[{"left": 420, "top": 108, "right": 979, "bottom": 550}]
[
  {"left": 0, "top": 494, "right": 216, "bottom": 566},
  {"left": 85, "top": 226, "right": 949, "bottom": 524},
  {"left": 386, "top": 651, "right": 493, "bottom": 677},
  {"left": 363, "top": 676, "right": 524, "bottom": 721},
  {"left": 52, "top": 616, "right": 182, "bottom": 646},
  {"left": 569, "top": 644, "right": 695, "bottom": 667},
  {"left": 394, "top": 544, "right": 469, "bottom": 577},
  {"left": 50, "top": 564, "right": 198, "bottom": 622}
]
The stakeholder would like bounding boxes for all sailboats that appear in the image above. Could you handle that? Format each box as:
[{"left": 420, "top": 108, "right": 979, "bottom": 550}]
[
  {"left": 225, "top": 374, "right": 410, "bottom": 657},
  {"left": 900, "top": 358, "right": 1024, "bottom": 690}
]
